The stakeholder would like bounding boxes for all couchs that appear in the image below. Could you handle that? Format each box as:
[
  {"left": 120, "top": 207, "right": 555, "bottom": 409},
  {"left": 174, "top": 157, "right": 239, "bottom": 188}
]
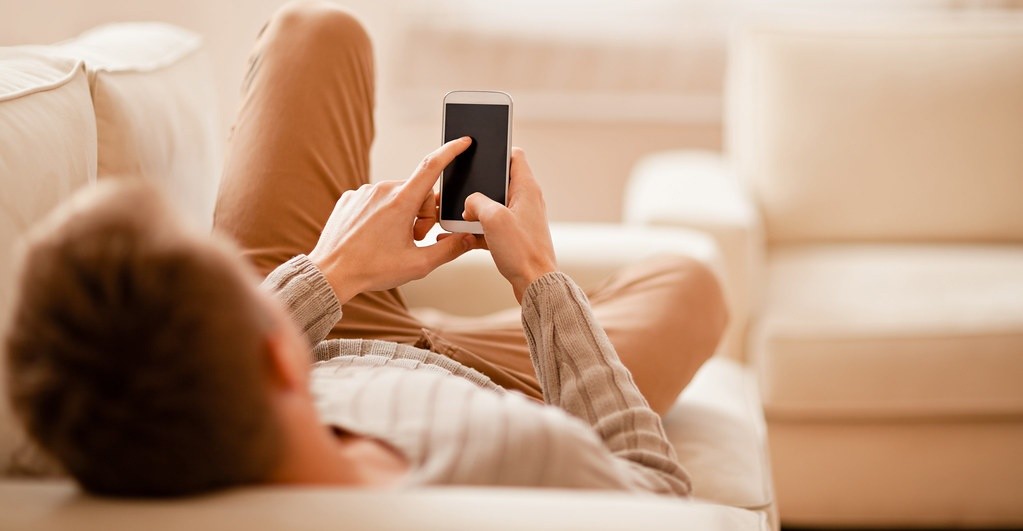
[
  {"left": 622, "top": 10, "right": 1023, "bottom": 524},
  {"left": 0, "top": 0, "right": 777, "bottom": 531}
]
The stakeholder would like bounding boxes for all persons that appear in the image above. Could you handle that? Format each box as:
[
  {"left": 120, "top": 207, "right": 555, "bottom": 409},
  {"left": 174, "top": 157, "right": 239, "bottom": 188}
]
[{"left": 5, "top": 1, "right": 730, "bottom": 504}]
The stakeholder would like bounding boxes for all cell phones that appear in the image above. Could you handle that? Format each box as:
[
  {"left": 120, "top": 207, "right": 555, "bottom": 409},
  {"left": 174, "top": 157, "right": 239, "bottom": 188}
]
[{"left": 439, "top": 91, "right": 514, "bottom": 234}]
